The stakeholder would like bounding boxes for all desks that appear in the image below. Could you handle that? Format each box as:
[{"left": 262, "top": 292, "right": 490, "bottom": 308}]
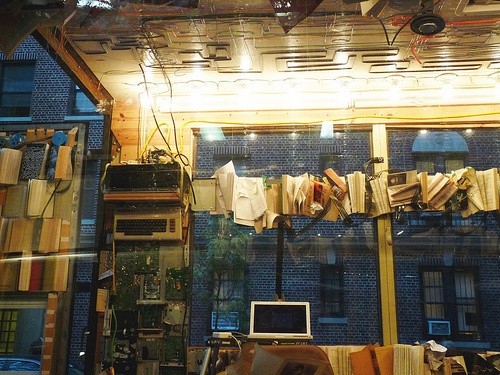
[{"left": 217, "top": 345, "right": 425, "bottom": 375}]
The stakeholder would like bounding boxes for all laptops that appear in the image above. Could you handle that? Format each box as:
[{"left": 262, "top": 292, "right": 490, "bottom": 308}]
[{"left": 245, "top": 301, "right": 313, "bottom": 340}]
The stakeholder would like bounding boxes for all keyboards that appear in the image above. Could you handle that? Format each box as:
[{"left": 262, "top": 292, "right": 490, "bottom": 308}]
[{"left": 112, "top": 207, "right": 181, "bottom": 241}]
